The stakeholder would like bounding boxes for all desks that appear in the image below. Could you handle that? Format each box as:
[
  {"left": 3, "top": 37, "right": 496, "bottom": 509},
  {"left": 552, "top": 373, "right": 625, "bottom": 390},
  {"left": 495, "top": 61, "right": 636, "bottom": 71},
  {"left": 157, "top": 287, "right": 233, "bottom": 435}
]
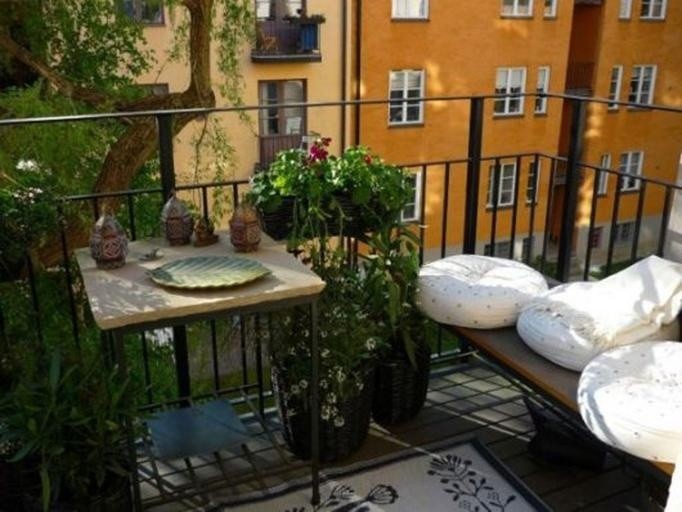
[{"left": 71, "top": 230, "right": 328, "bottom": 511}]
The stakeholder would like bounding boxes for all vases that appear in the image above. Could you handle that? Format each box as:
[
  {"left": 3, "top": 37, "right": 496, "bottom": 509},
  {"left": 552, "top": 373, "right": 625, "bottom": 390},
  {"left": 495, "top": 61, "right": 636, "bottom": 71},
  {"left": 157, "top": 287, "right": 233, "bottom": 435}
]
[{"left": 266, "top": 349, "right": 430, "bottom": 467}]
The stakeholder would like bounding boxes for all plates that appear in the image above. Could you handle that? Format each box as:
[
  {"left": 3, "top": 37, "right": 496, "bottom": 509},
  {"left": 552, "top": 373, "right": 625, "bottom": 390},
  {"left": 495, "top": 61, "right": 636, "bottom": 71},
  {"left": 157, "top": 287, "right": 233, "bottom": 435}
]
[{"left": 145, "top": 254, "right": 273, "bottom": 289}]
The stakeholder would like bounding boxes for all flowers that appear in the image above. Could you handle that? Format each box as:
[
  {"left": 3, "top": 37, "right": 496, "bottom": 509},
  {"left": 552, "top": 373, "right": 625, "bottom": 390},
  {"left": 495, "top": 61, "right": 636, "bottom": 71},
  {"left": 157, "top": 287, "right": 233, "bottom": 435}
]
[{"left": 244, "top": 130, "right": 432, "bottom": 427}]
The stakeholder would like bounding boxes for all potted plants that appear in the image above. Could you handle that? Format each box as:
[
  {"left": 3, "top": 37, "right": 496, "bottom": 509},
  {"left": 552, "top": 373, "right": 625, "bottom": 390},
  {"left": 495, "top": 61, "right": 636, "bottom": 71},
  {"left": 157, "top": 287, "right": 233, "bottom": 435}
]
[{"left": 1, "top": 345, "right": 156, "bottom": 512}]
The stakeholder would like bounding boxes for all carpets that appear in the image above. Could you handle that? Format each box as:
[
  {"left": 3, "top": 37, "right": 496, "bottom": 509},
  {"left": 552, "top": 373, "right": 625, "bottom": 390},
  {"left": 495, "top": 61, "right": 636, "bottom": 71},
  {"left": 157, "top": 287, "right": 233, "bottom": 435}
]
[{"left": 190, "top": 432, "right": 552, "bottom": 510}]
